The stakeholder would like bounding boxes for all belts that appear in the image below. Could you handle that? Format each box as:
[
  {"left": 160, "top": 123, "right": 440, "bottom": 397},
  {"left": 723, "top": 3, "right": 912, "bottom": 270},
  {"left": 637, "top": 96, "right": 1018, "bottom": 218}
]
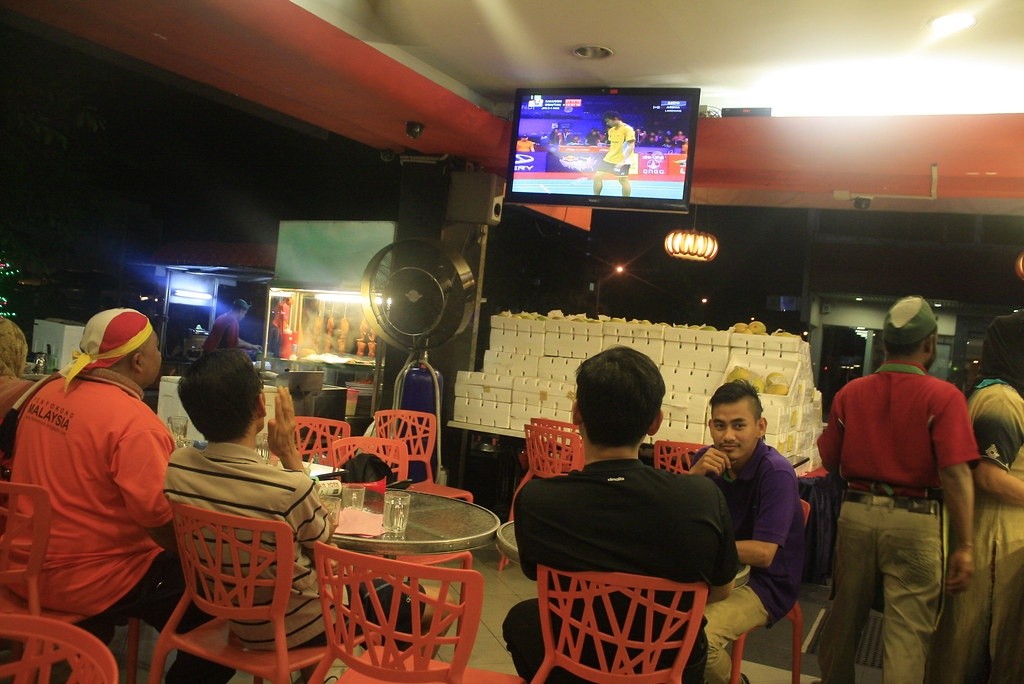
[{"left": 843, "top": 491, "right": 936, "bottom": 514}]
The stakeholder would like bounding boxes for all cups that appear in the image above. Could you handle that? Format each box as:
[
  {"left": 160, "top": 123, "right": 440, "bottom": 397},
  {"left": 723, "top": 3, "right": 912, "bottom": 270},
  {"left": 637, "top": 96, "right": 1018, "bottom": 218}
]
[
  {"left": 381, "top": 491, "right": 411, "bottom": 533},
  {"left": 321, "top": 496, "right": 342, "bottom": 526},
  {"left": 342, "top": 485, "right": 366, "bottom": 512},
  {"left": 167, "top": 416, "right": 188, "bottom": 438},
  {"left": 177, "top": 437, "right": 194, "bottom": 448},
  {"left": 36, "top": 354, "right": 45, "bottom": 374},
  {"left": 255, "top": 432, "right": 269, "bottom": 463}
]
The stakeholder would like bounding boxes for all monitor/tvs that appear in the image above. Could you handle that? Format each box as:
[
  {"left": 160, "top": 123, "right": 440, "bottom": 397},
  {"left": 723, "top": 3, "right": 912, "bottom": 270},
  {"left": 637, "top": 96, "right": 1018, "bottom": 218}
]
[{"left": 505, "top": 87, "right": 701, "bottom": 212}]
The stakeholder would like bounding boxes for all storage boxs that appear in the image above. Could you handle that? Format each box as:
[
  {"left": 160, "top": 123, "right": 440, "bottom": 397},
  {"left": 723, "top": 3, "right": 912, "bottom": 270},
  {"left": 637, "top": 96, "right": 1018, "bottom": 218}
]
[
  {"left": 156, "top": 375, "right": 278, "bottom": 445},
  {"left": 448, "top": 312, "right": 828, "bottom": 480}
]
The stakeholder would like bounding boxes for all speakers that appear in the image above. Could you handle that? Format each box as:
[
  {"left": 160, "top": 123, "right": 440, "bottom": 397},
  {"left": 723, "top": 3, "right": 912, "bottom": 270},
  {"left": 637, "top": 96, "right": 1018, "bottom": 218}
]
[{"left": 445, "top": 171, "right": 505, "bottom": 225}]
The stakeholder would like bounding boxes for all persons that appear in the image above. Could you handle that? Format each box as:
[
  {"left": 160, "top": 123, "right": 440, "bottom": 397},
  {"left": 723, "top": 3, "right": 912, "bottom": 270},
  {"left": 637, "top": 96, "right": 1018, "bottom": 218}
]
[
  {"left": 550, "top": 128, "right": 688, "bottom": 154},
  {"left": 518, "top": 134, "right": 535, "bottom": 152},
  {"left": 163, "top": 348, "right": 456, "bottom": 684},
  {"left": 502, "top": 346, "right": 740, "bottom": 684},
  {"left": 0, "top": 316, "right": 36, "bottom": 505},
  {"left": 926, "top": 309, "right": 1024, "bottom": 684},
  {"left": 0, "top": 307, "right": 237, "bottom": 684},
  {"left": 201, "top": 299, "right": 262, "bottom": 357},
  {"left": 272, "top": 298, "right": 290, "bottom": 356},
  {"left": 814, "top": 294, "right": 980, "bottom": 684},
  {"left": 686, "top": 377, "right": 805, "bottom": 683},
  {"left": 593, "top": 111, "right": 636, "bottom": 197}
]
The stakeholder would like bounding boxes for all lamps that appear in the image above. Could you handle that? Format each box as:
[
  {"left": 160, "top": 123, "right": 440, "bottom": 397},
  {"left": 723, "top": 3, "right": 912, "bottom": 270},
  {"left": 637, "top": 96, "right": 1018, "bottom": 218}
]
[{"left": 665, "top": 205, "right": 719, "bottom": 262}]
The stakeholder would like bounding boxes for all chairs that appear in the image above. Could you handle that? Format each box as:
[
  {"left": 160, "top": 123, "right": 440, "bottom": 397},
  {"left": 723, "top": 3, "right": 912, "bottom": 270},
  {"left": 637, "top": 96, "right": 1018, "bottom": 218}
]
[{"left": 0, "top": 409, "right": 827, "bottom": 684}]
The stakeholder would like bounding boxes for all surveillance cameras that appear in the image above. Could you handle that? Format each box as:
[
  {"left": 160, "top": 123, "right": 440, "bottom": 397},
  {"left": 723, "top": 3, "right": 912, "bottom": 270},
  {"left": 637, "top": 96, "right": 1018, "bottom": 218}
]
[{"left": 406, "top": 121, "right": 423, "bottom": 137}]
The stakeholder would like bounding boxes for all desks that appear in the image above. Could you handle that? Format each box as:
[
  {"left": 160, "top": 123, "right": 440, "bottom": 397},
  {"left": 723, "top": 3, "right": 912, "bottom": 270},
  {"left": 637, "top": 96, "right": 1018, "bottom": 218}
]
[
  {"left": 318, "top": 486, "right": 502, "bottom": 581},
  {"left": 494, "top": 517, "right": 752, "bottom": 589}
]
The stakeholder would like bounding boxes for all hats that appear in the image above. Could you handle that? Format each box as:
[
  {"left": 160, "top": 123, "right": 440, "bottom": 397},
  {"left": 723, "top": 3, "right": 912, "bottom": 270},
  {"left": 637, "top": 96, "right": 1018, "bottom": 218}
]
[{"left": 883, "top": 296, "right": 936, "bottom": 345}]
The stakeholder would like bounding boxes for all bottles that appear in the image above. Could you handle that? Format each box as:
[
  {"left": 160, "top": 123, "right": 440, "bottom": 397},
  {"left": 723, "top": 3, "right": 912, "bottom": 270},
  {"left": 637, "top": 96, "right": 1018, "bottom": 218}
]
[
  {"left": 288, "top": 344, "right": 299, "bottom": 372},
  {"left": 45, "top": 343, "right": 54, "bottom": 374}
]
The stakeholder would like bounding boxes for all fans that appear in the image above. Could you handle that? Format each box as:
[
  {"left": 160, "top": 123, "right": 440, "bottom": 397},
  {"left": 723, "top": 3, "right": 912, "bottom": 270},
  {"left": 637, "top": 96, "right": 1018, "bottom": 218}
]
[{"left": 357, "top": 234, "right": 475, "bottom": 485}]
